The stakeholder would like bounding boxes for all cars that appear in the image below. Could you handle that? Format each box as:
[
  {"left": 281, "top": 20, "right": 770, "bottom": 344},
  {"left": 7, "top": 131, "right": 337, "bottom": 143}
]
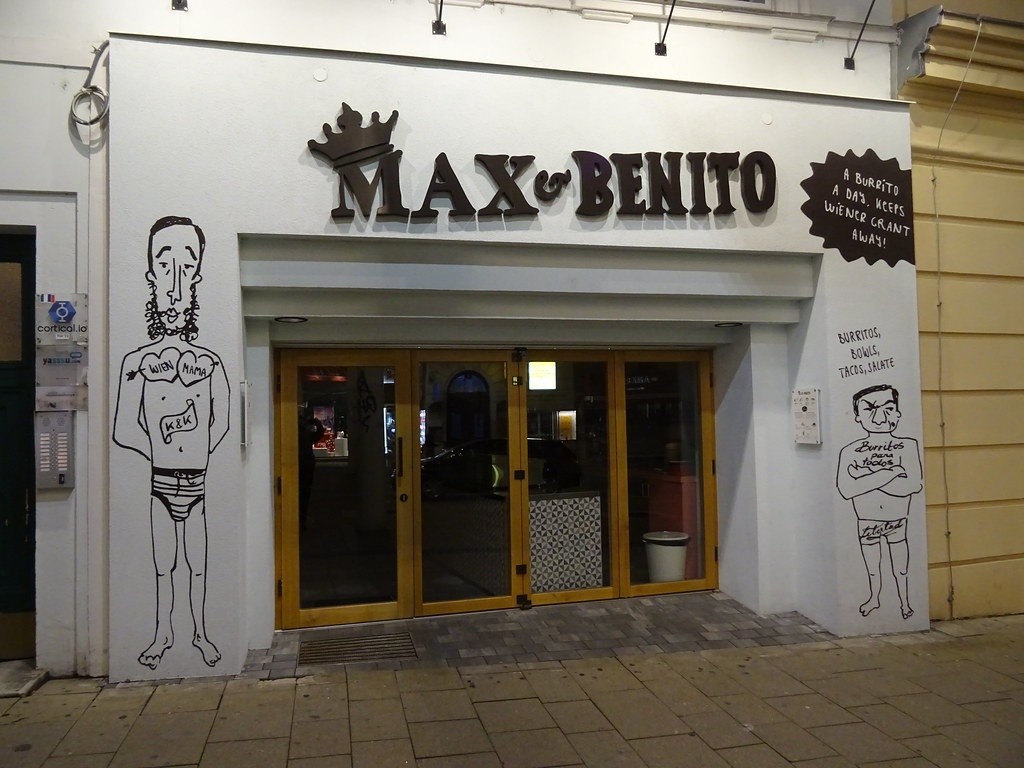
[{"left": 390, "top": 435, "right": 591, "bottom": 499}]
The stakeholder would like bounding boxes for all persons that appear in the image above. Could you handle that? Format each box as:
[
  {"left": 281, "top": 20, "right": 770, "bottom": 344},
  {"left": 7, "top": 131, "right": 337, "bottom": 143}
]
[{"left": 298, "top": 415, "right": 324, "bottom": 530}]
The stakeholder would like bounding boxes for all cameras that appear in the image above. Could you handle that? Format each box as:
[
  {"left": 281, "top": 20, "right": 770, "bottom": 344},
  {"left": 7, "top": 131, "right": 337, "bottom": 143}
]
[{"left": 308, "top": 419, "right": 315, "bottom": 425}]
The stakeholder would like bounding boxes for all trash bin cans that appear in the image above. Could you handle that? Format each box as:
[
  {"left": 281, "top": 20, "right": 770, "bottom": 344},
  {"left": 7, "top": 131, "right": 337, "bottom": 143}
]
[{"left": 642, "top": 530, "right": 691, "bottom": 583}]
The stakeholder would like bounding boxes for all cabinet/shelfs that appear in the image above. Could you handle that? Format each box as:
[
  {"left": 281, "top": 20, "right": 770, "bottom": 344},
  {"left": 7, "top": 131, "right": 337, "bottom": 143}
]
[{"left": 643, "top": 470, "right": 699, "bottom": 579}]
